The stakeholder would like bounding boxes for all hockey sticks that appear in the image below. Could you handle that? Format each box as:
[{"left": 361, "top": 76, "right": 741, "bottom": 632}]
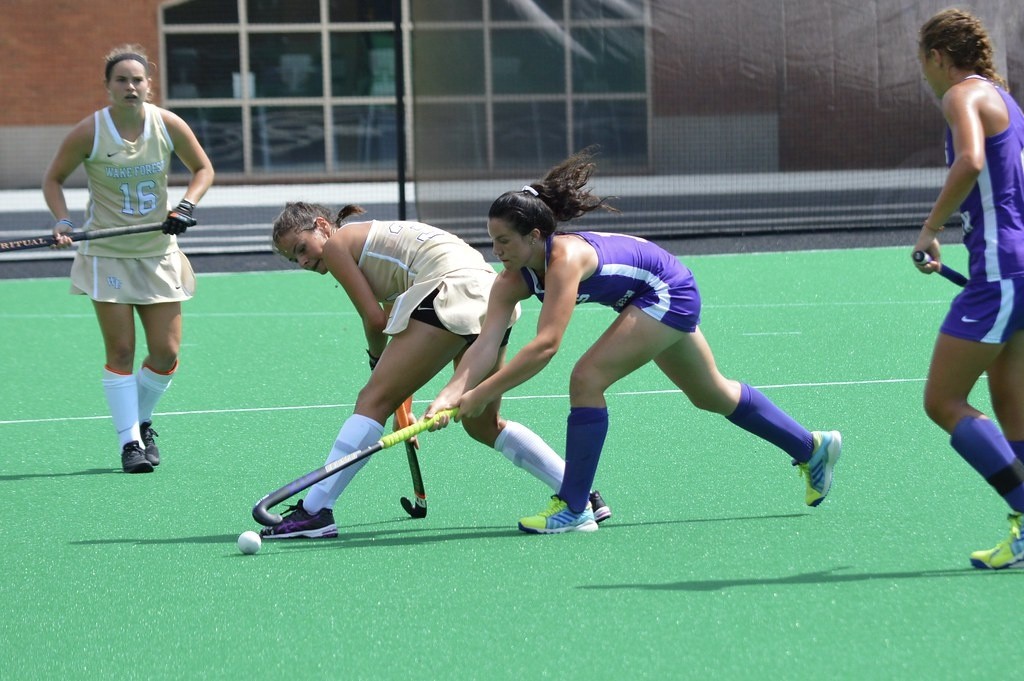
[
  {"left": 252, "top": 406, "right": 458, "bottom": 529},
  {"left": 912, "top": 249, "right": 970, "bottom": 288},
  {"left": 0, "top": 218, "right": 197, "bottom": 252},
  {"left": 399, "top": 400, "right": 429, "bottom": 518}
]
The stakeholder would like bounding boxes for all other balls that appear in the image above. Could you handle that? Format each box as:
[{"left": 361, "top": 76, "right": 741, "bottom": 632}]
[{"left": 238, "top": 531, "right": 262, "bottom": 555}]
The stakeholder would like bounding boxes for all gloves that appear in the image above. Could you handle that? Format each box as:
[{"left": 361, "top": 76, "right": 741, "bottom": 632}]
[{"left": 162, "top": 199, "right": 195, "bottom": 236}]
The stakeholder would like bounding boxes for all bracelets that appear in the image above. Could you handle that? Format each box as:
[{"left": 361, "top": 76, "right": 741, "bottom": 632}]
[
  {"left": 55, "top": 220, "right": 74, "bottom": 228},
  {"left": 924, "top": 219, "right": 945, "bottom": 232}
]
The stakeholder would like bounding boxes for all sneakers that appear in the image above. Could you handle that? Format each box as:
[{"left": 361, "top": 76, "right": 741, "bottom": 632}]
[
  {"left": 517, "top": 494, "right": 599, "bottom": 534},
  {"left": 120, "top": 440, "right": 154, "bottom": 473},
  {"left": 138, "top": 419, "right": 160, "bottom": 466},
  {"left": 258, "top": 498, "right": 339, "bottom": 540},
  {"left": 791, "top": 430, "right": 841, "bottom": 507},
  {"left": 589, "top": 490, "right": 610, "bottom": 523},
  {"left": 968, "top": 512, "right": 1024, "bottom": 571}
]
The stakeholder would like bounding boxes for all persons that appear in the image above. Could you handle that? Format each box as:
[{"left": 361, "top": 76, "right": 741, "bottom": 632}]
[
  {"left": 913, "top": 6, "right": 1023, "bottom": 570},
  {"left": 42, "top": 45, "right": 215, "bottom": 476},
  {"left": 421, "top": 145, "right": 844, "bottom": 534},
  {"left": 258, "top": 201, "right": 612, "bottom": 540}
]
